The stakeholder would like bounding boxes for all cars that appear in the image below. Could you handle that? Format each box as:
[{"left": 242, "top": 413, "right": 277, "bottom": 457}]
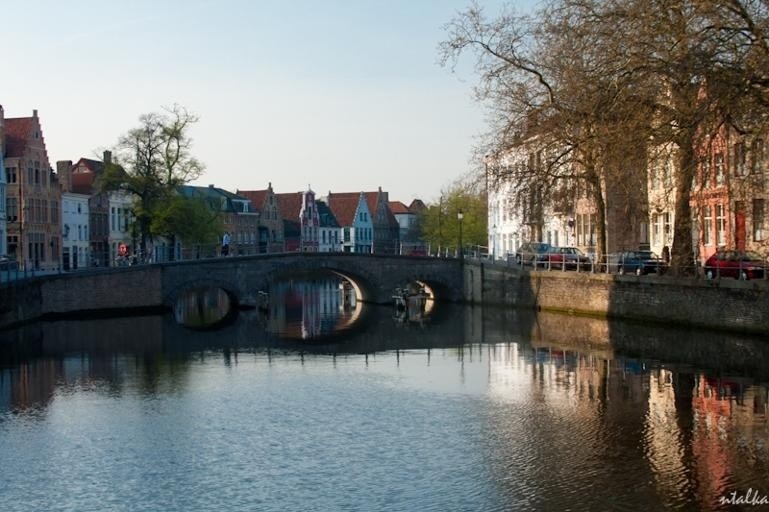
[
  {"left": 542, "top": 247, "right": 590, "bottom": 270},
  {"left": 702, "top": 248, "right": 769, "bottom": 279},
  {"left": 515, "top": 242, "right": 556, "bottom": 268},
  {"left": 616, "top": 250, "right": 667, "bottom": 275},
  {"left": 0, "top": 255, "right": 20, "bottom": 271}
]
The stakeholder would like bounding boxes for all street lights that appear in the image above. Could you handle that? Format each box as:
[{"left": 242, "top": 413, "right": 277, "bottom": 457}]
[{"left": 455, "top": 209, "right": 465, "bottom": 258}]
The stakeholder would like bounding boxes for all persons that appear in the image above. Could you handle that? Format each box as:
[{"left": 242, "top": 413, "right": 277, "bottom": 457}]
[
  {"left": 86, "top": 248, "right": 155, "bottom": 268},
  {"left": 221, "top": 230, "right": 233, "bottom": 256}
]
